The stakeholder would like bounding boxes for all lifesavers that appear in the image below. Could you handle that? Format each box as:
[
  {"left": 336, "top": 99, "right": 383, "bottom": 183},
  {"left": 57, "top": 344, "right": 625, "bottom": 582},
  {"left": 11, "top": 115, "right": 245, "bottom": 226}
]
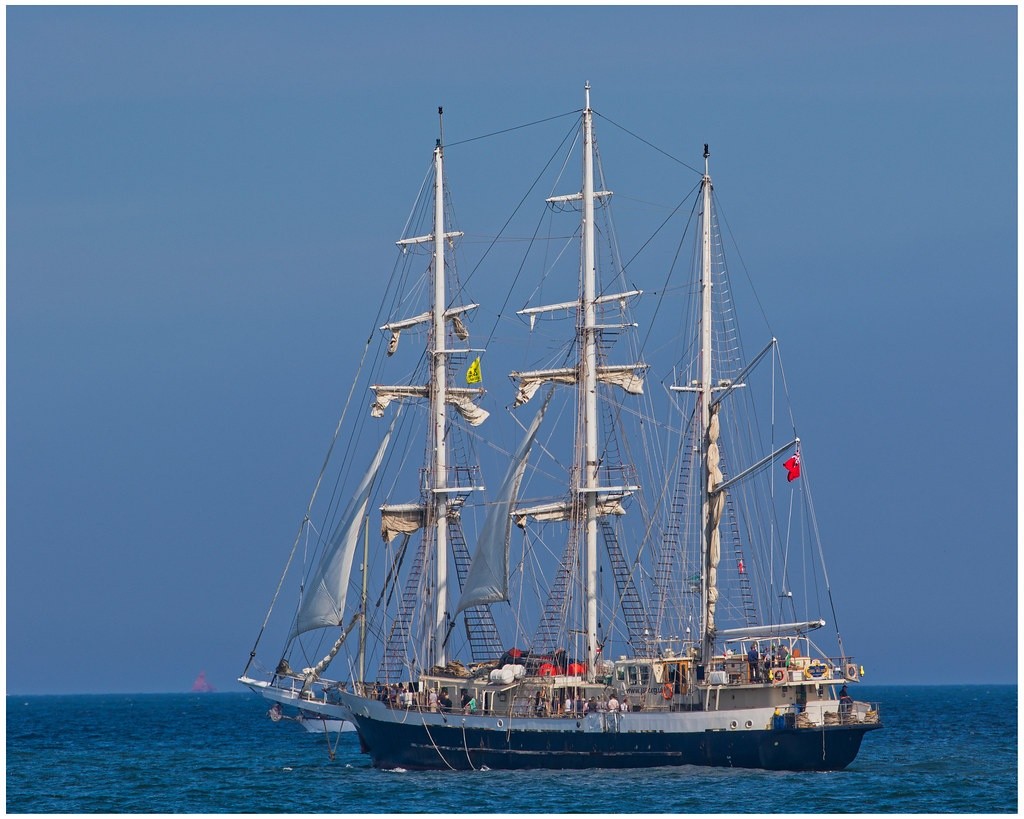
[
  {"left": 661, "top": 684, "right": 673, "bottom": 700},
  {"left": 771, "top": 667, "right": 787, "bottom": 685},
  {"left": 845, "top": 663, "right": 858, "bottom": 680}
]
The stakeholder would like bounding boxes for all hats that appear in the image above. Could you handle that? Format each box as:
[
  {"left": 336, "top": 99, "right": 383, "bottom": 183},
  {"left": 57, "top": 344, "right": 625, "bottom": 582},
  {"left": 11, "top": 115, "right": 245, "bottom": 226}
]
[{"left": 843, "top": 685, "right": 848, "bottom": 688}]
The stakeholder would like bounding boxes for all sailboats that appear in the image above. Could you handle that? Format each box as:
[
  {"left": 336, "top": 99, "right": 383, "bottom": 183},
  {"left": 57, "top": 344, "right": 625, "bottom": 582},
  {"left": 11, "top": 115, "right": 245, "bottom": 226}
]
[
  {"left": 238, "top": 78, "right": 885, "bottom": 772},
  {"left": 262, "top": 516, "right": 370, "bottom": 735}
]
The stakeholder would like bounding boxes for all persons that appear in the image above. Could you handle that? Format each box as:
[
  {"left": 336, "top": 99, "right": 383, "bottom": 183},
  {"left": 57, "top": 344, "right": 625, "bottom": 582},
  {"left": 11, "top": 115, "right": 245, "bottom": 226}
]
[{"left": 374, "top": 644, "right": 855, "bottom": 722}]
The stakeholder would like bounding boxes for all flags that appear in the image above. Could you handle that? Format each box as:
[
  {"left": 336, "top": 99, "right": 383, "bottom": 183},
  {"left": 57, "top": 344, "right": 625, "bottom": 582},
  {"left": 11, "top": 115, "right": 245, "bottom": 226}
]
[
  {"left": 465, "top": 357, "right": 482, "bottom": 383},
  {"left": 783, "top": 447, "right": 800, "bottom": 482}
]
